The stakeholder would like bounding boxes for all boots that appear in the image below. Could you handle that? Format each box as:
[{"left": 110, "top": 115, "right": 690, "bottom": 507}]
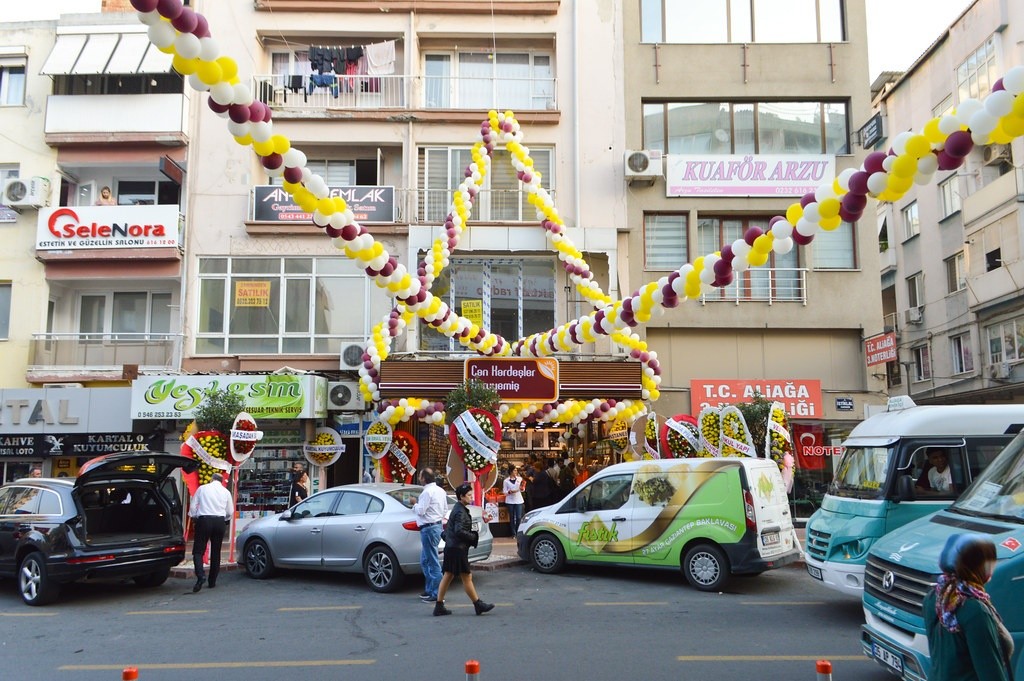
[
  {"left": 474, "top": 599, "right": 494, "bottom": 614},
  {"left": 433, "top": 600, "right": 452, "bottom": 616}
]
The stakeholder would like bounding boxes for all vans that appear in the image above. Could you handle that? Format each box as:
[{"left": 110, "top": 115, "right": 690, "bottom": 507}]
[
  {"left": 803, "top": 395, "right": 1024, "bottom": 598},
  {"left": 857, "top": 425, "right": 1023, "bottom": 681},
  {"left": 515, "top": 455, "right": 801, "bottom": 593}
]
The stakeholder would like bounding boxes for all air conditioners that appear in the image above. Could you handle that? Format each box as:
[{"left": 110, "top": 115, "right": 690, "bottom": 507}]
[
  {"left": 1, "top": 178, "right": 48, "bottom": 209},
  {"left": 622, "top": 150, "right": 663, "bottom": 180},
  {"left": 983, "top": 143, "right": 1008, "bottom": 167},
  {"left": 905, "top": 307, "right": 919, "bottom": 324},
  {"left": 991, "top": 363, "right": 1009, "bottom": 378},
  {"left": 340, "top": 342, "right": 368, "bottom": 370},
  {"left": 327, "top": 382, "right": 366, "bottom": 410}
]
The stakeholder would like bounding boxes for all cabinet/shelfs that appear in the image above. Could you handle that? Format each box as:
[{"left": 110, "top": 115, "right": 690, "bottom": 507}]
[{"left": 585, "top": 437, "right": 610, "bottom": 472}]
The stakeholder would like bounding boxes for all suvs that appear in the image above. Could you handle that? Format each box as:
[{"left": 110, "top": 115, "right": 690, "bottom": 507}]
[{"left": 0, "top": 449, "right": 203, "bottom": 607}]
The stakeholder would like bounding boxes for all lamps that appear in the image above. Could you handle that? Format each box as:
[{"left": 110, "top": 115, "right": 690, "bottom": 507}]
[{"left": 504, "top": 428, "right": 537, "bottom": 433}]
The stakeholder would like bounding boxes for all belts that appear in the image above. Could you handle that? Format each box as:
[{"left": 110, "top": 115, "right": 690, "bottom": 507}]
[
  {"left": 198, "top": 515, "right": 223, "bottom": 518},
  {"left": 419, "top": 521, "right": 442, "bottom": 530}
]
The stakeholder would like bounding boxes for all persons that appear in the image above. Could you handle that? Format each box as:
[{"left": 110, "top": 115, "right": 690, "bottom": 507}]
[
  {"left": 922, "top": 533, "right": 1013, "bottom": 681},
  {"left": 916, "top": 446, "right": 953, "bottom": 493},
  {"left": 521, "top": 445, "right": 579, "bottom": 511},
  {"left": 291, "top": 471, "right": 307, "bottom": 507},
  {"left": 30, "top": 468, "right": 41, "bottom": 478},
  {"left": 95, "top": 186, "right": 117, "bottom": 205},
  {"left": 411, "top": 468, "right": 448, "bottom": 603},
  {"left": 502, "top": 465, "right": 525, "bottom": 539},
  {"left": 187, "top": 473, "right": 234, "bottom": 592},
  {"left": 363, "top": 467, "right": 371, "bottom": 483},
  {"left": 294, "top": 463, "right": 310, "bottom": 497},
  {"left": 432, "top": 484, "right": 495, "bottom": 616}
]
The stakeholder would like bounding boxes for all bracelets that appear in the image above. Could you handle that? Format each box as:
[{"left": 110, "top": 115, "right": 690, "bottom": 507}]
[{"left": 510, "top": 491, "right": 512, "bottom": 493}]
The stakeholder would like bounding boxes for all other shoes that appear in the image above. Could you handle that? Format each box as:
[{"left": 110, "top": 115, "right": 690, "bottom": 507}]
[
  {"left": 208, "top": 581, "right": 216, "bottom": 588},
  {"left": 193, "top": 576, "right": 206, "bottom": 591}
]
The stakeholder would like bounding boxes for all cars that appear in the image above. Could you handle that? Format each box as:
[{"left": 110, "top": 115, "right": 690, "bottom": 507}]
[{"left": 235, "top": 480, "right": 494, "bottom": 595}]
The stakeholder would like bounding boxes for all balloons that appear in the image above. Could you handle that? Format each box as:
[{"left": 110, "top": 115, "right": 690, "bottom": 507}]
[{"left": 130, "top": 0, "right": 1024, "bottom": 440}]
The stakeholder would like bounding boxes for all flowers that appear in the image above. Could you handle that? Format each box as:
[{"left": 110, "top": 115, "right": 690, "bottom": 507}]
[
  {"left": 191, "top": 435, "right": 227, "bottom": 485},
  {"left": 387, "top": 436, "right": 414, "bottom": 484},
  {"left": 455, "top": 413, "right": 495, "bottom": 471},
  {"left": 234, "top": 420, "right": 256, "bottom": 454},
  {"left": 308, "top": 433, "right": 337, "bottom": 465},
  {"left": 366, "top": 423, "right": 389, "bottom": 453},
  {"left": 608, "top": 408, "right": 796, "bottom": 478}
]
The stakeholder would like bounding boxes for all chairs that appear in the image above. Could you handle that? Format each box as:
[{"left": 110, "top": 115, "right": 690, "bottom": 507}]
[{"left": 83, "top": 489, "right": 132, "bottom": 535}]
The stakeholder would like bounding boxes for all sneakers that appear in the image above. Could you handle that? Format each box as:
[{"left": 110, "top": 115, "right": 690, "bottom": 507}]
[
  {"left": 419, "top": 592, "right": 430, "bottom": 598},
  {"left": 422, "top": 596, "right": 438, "bottom": 603}
]
